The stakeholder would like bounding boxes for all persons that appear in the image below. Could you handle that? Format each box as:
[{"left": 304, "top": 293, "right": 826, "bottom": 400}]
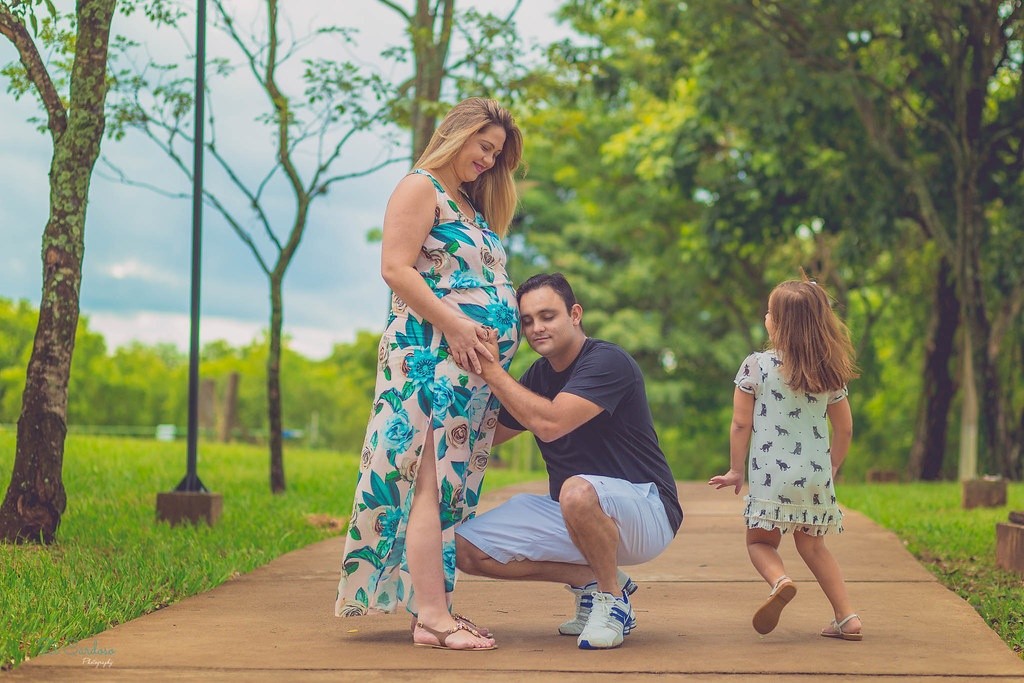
[
  {"left": 707, "top": 280, "right": 865, "bottom": 641},
  {"left": 333, "top": 97, "right": 524, "bottom": 651},
  {"left": 448, "top": 274, "right": 685, "bottom": 654}
]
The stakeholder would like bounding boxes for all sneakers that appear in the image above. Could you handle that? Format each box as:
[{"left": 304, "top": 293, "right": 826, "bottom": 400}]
[
  {"left": 558, "top": 568, "right": 638, "bottom": 636},
  {"left": 577, "top": 589, "right": 637, "bottom": 649}
]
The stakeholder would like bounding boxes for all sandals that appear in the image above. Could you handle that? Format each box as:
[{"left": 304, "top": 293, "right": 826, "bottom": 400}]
[
  {"left": 413, "top": 620, "right": 498, "bottom": 651},
  {"left": 411, "top": 613, "right": 493, "bottom": 638},
  {"left": 821, "top": 614, "right": 863, "bottom": 640},
  {"left": 752, "top": 576, "right": 797, "bottom": 635}
]
